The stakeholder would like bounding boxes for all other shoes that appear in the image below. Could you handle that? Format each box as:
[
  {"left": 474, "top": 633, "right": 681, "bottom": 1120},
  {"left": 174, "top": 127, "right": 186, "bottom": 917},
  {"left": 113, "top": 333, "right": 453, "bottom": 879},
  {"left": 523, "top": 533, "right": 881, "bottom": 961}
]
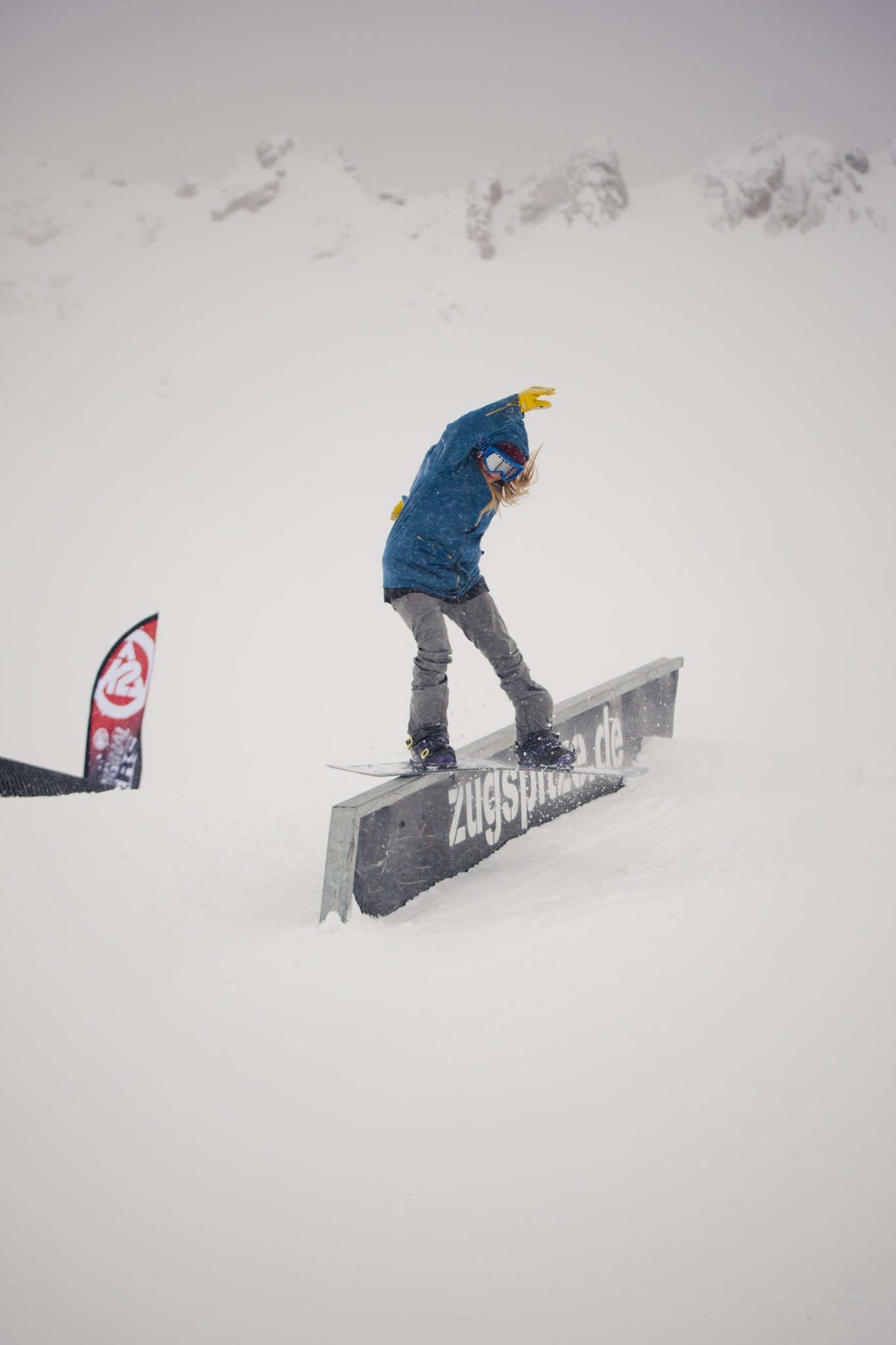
[
  {"left": 513, "top": 729, "right": 576, "bottom": 770},
  {"left": 404, "top": 725, "right": 458, "bottom": 769}
]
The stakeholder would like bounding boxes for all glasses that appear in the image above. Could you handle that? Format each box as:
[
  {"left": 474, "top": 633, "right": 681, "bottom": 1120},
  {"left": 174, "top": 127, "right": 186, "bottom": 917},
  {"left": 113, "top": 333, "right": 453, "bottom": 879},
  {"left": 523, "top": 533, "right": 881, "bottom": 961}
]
[{"left": 479, "top": 436, "right": 524, "bottom": 481}]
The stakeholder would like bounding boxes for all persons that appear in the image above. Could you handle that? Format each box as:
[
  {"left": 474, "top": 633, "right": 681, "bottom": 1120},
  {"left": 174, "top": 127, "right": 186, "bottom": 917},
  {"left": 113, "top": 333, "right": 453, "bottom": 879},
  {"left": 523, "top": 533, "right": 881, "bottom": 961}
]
[{"left": 381, "top": 384, "right": 581, "bottom": 772}]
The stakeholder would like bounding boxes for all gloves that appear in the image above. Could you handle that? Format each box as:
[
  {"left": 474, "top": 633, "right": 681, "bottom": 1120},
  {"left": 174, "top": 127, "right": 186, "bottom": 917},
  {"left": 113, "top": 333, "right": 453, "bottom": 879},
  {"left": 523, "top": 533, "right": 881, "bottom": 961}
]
[
  {"left": 390, "top": 500, "right": 403, "bottom": 521},
  {"left": 518, "top": 385, "right": 556, "bottom": 413}
]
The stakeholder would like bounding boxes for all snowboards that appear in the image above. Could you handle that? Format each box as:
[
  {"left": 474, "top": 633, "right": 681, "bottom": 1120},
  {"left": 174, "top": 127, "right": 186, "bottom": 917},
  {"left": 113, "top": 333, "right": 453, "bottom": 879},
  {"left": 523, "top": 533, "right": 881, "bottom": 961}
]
[{"left": 323, "top": 758, "right": 650, "bottom": 777}]
